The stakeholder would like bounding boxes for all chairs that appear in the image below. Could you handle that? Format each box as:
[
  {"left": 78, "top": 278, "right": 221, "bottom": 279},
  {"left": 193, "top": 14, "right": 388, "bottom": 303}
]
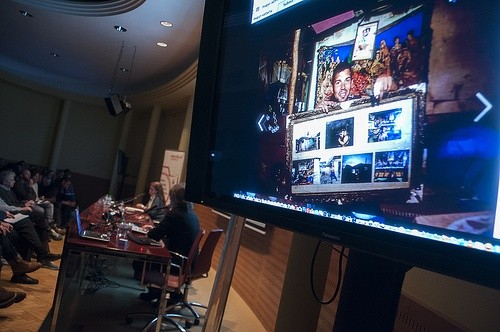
[{"left": 127, "top": 228, "right": 224, "bottom": 332}]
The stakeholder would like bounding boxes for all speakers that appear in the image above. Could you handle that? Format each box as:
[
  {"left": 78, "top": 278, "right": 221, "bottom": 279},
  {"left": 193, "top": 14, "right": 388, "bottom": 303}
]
[{"left": 104, "top": 94, "right": 123, "bottom": 116}]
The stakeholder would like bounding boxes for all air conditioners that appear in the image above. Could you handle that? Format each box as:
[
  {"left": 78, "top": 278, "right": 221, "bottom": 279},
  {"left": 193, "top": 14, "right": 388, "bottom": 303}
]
[{"left": 158, "top": 148, "right": 186, "bottom": 204}]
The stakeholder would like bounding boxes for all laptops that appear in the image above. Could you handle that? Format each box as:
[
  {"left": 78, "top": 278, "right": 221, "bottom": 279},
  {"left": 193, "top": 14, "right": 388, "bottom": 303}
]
[{"left": 74, "top": 206, "right": 110, "bottom": 242}]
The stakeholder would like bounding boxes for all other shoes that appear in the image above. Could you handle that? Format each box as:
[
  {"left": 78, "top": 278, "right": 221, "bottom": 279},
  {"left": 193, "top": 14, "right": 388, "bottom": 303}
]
[
  {"left": 0, "top": 288, "right": 26, "bottom": 309},
  {"left": 139, "top": 290, "right": 162, "bottom": 301},
  {"left": 166, "top": 296, "right": 183, "bottom": 306},
  {"left": 11, "top": 260, "right": 42, "bottom": 284},
  {"left": 51, "top": 228, "right": 67, "bottom": 241},
  {"left": 35, "top": 253, "right": 62, "bottom": 270}
]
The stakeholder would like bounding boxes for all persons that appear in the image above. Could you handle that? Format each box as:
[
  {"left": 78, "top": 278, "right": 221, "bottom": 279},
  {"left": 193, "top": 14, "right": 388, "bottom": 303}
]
[
  {"left": 316, "top": 32, "right": 417, "bottom": 113},
  {"left": 131, "top": 184, "right": 199, "bottom": 309},
  {"left": 136, "top": 182, "right": 166, "bottom": 221},
  {"left": 0, "top": 160, "right": 77, "bottom": 308}
]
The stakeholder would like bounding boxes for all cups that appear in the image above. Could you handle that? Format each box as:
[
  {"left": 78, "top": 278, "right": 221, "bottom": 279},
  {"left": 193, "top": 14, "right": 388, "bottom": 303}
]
[{"left": 115, "top": 224, "right": 132, "bottom": 248}]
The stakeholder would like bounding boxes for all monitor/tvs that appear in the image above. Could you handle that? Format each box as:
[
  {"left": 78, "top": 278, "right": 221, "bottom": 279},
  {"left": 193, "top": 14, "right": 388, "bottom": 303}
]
[{"left": 182, "top": 0, "right": 500, "bottom": 290}]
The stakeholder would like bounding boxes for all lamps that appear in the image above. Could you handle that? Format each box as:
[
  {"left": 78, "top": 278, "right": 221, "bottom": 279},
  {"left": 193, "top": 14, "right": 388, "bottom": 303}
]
[{"left": 105, "top": 89, "right": 131, "bottom": 122}]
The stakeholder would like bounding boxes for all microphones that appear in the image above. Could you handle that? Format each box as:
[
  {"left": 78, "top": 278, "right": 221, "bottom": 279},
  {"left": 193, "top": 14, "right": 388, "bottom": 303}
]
[
  {"left": 135, "top": 191, "right": 148, "bottom": 199},
  {"left": 126, "top": 205, "right": 158, "bottom": 223}
]
